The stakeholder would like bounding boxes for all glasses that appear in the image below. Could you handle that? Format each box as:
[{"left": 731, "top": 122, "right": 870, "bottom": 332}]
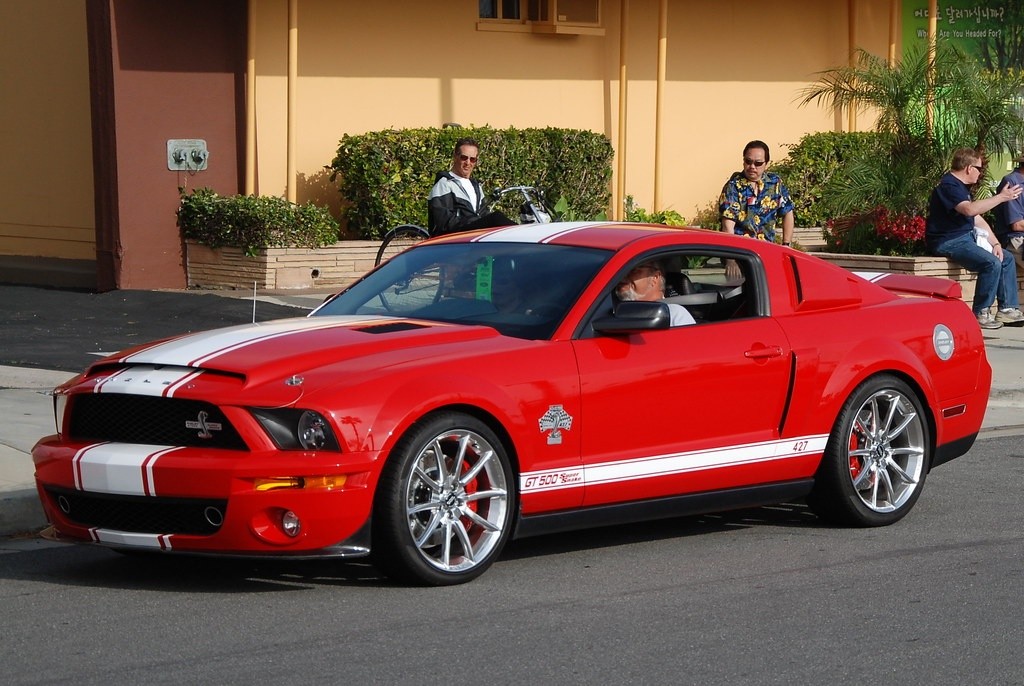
[
  {"left": 632, "top": 265, "right": 659, "bottom": 274},
  {"left": 973, "top": 165, "right": 982, "bottom": 172},
  {"left": 745, "top": 158, "right": 766, "bottom": 166},
  {"left": 460, "top": 154, "right": 476, "bottom": 163}
]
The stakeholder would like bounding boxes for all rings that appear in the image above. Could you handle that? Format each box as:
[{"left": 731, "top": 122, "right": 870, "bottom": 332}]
[{"left": 1015, "top": 194, "right": 1016, "bottom": 197}]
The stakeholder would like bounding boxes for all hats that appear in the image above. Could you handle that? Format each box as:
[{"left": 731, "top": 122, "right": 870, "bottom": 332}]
[{"left": 1012, "top": 146, "right": 1024, "bottom": 162}]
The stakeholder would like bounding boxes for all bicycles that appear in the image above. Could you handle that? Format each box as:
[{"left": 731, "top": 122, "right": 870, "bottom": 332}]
[{"left": 374, "top": 184, "right": 558, "bottom": 318}]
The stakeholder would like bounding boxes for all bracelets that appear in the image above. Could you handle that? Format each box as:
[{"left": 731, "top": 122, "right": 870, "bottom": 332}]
[{"left": 993, "top": 243, "right": 1000, "bottom": 249}]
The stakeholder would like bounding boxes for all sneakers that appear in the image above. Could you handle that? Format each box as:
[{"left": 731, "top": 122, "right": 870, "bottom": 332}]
[
  {"left": 978, "top": 307, "right": 1003, "bottom": 328},
  {"left": 995, "top": 311, "right": 1024, "bottom": 322}
]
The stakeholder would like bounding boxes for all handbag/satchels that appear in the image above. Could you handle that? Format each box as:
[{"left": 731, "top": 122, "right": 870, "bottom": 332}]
[{"left": 974, "top": 227, "right": 992, "bottom": 253}]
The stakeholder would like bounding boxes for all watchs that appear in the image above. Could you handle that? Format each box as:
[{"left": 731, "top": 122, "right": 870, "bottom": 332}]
[{"left": 781, "top": 242, "right": 792, "bottom": 247}]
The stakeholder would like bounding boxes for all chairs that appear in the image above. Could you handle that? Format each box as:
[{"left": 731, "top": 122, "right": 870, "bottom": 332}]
[{"left": 663, "top": 270, "right": 709, "bottom": 321}]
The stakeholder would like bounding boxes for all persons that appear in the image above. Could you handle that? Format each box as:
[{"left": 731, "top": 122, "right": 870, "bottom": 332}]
[
  {"left": 925, "top": 148, "right": 1024, "bottom": 329},
  {"left": 488, "top": 265, "right": 541, "bottom": 316},
  {"left": 993, "top": 152, "right": 1024, "bottom": 269},
  {"left": 718, "top": 139, "right": 795, "bottom": 279},
  {"left": 427, "top": 137, "right": 519, "bottom": 240},
  {"left": 614, "top": 255, "right": 697, "bottom": 327}
]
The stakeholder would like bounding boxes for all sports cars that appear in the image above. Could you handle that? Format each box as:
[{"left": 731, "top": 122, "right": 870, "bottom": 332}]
[{"left": 29, "top": 219, "right": 994, "bottom": 587}]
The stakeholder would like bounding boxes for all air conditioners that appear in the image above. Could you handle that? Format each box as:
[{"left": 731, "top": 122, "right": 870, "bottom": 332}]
[{"left": 528, "top": 0, "right": 602, "bottom": 27}]
